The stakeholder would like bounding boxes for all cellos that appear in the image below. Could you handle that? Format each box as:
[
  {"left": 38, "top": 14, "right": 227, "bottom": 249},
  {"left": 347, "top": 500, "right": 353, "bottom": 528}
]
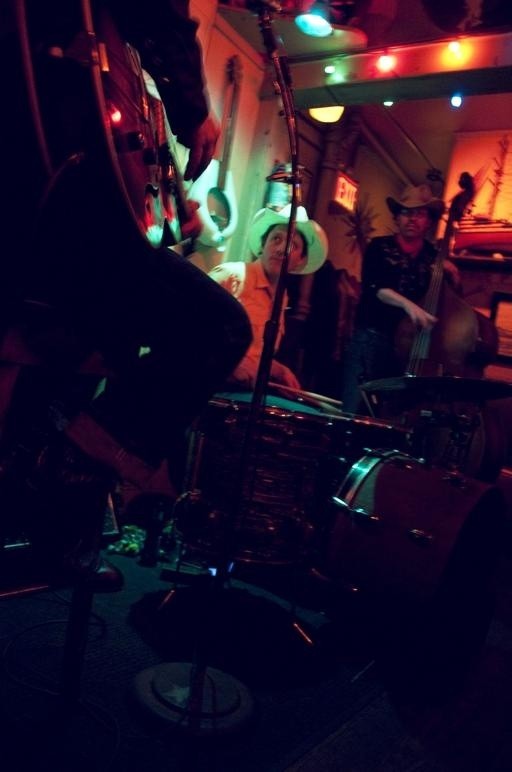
[{"left": 391, "top": 172, "right": 489, "bottom": 479}]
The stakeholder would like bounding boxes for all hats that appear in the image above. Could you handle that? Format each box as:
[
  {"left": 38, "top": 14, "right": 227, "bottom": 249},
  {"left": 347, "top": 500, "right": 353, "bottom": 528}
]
[
  {"left": 244, "top": 199, "right": 330, "bottom": 275},
  {"left": 386, "top": 184, "right": 445, "bottom": 224}
]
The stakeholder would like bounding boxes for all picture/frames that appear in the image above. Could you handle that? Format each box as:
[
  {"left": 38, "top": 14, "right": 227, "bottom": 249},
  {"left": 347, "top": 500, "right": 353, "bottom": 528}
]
[{"left": 489, "top": 291, "right": 511, "bottom": 365}]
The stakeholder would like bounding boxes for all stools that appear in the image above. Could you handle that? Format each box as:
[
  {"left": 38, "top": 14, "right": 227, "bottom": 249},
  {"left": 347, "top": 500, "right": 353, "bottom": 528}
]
[{"left": 1, "top": 323, "right": 128, "bottom": 702}]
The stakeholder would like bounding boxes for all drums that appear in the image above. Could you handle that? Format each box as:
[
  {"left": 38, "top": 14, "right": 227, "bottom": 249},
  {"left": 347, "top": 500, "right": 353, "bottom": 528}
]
[
  {"left": 174, "top": 396, "right": 333, "bottom": 567},
  {"left": 310, "top": 406, "right": 414, "bottom": 506},
  {"left": 313, "top": 443, "right": 505, "bottom": 650}
]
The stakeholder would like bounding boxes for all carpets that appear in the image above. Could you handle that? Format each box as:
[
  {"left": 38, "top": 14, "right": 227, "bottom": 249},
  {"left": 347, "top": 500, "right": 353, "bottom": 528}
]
[{"left": 1, "top": 518, "right": 386, "bottom": 769}]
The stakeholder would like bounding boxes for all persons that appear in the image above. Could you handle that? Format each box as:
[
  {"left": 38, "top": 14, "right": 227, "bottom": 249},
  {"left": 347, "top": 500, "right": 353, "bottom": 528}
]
[
  {"left": 202, "top": 199, "right": 334, "bottom": 401},
  {"left": 335, "top": 182, "right": 500, "bottom": 431},
  {"left": 4, "top": 1, "right": 253, "bottom": 594}
]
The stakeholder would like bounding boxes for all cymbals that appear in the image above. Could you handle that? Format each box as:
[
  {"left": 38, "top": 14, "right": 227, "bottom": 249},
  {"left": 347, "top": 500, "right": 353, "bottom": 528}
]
[{"left": 364, "top": 377, "right": 511, "bottom": 400}]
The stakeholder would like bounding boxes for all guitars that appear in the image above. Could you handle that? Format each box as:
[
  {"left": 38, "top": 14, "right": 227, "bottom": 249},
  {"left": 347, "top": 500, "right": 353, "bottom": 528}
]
[{"left": 44, "top": 11, "right": 198, "bottom": 261}]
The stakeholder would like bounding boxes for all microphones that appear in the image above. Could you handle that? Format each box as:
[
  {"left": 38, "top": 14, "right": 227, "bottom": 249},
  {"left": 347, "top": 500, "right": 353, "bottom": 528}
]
[{"left": 218, "top": 0, "right": 277, "bottom": 15}]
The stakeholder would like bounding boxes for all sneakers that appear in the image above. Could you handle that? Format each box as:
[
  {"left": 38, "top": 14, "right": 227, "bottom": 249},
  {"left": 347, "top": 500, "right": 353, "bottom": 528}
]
[{"left": 64, "top": 408, "right": 154, "bottom": 491}]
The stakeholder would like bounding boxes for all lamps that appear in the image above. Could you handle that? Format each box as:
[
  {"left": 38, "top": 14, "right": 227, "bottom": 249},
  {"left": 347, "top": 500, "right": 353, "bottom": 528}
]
[{"left": 293, "top": 1, "right": 336, "bottom": 38}]
